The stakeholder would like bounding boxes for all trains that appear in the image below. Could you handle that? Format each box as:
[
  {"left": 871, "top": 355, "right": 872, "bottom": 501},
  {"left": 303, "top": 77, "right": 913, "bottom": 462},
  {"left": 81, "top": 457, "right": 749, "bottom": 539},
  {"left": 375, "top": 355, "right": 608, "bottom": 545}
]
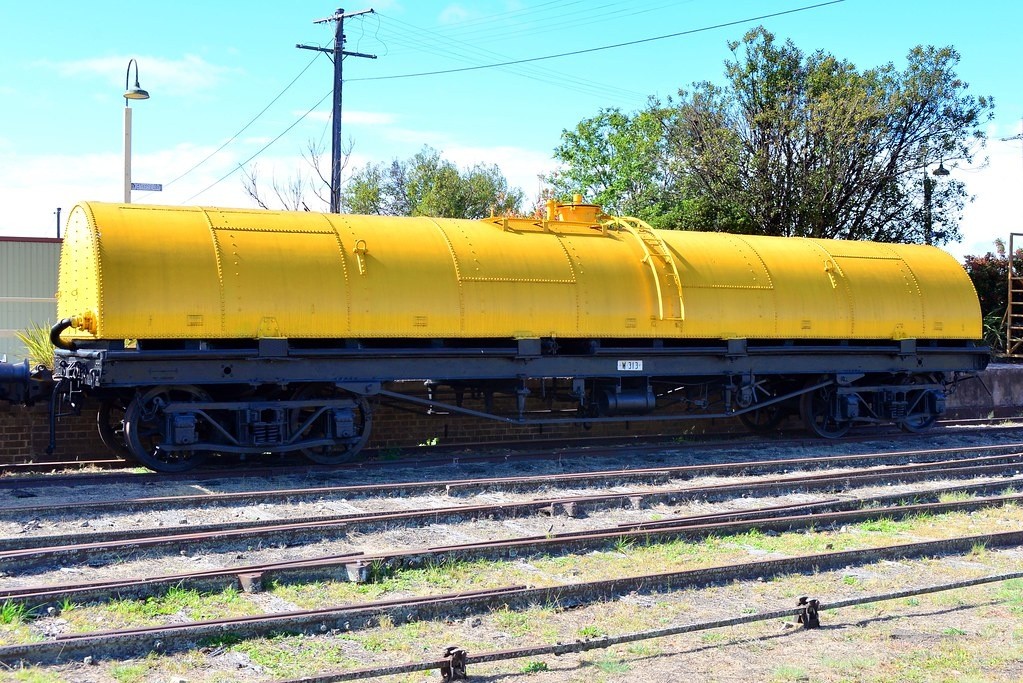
[{"left": 42, "top": 184, "right": 991, "bottom": 473}]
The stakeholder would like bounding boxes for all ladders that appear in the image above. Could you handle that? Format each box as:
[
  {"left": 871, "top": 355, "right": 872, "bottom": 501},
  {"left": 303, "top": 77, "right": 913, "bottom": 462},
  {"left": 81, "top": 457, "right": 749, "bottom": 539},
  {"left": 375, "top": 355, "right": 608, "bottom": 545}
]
[{"left": 997, "top": 232, "right": 1023, "bottom": 358}]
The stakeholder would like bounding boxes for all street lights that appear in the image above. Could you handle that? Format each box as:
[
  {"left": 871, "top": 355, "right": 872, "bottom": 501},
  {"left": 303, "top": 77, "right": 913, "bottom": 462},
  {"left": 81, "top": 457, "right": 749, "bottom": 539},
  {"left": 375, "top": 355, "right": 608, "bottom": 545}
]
[
  {"left": 119, "top": 57, "right": 150, "bottom": 205},
  {"left": 921, "top": 146, "right": 951, "bottom": 246}
]
